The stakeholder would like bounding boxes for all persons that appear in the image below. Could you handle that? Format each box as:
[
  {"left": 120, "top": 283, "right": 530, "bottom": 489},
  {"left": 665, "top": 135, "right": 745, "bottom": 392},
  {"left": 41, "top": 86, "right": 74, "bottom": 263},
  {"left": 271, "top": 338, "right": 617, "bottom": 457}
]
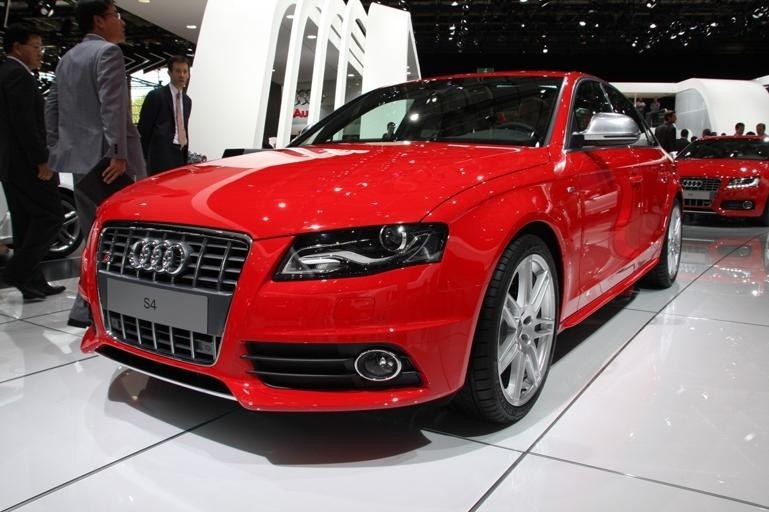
[
  {"left": 45, "top": 0, "right": 147, "bottom": 329},
  {"left": 653, "top": 111, "right": 769, "bottom": 159},
  {"left": 382, "top": 122, "right": 396, "bottom": 140},
  {"left": 137, "top": 55, "right": 193, "bottom": 178},
  {"left": 0, "top": 21, "right": 66, "bottom": 301}
]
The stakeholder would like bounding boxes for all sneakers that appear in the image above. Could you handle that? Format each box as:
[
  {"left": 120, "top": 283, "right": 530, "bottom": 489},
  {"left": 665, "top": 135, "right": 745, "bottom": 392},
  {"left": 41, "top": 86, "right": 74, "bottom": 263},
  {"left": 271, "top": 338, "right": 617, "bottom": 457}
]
[
  {"left": 67, "top": 312, "right": 93, "bottom": 328},
  {"left": 0, "top": 265, "right": 66, "bottom": 303}
]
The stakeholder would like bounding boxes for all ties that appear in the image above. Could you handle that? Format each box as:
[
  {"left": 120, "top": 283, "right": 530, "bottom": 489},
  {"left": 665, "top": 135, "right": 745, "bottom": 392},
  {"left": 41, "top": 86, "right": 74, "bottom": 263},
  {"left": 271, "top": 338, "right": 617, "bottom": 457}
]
[{"left": 176, "top": 89, "right": 189, "bottom": 151}]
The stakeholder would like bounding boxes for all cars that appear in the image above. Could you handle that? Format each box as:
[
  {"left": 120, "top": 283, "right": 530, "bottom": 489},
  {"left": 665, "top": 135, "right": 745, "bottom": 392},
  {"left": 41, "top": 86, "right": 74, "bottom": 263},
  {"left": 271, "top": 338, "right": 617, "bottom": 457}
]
[
  {"left": 0, "top": 173, "right": 89, "bottom": 260},
  {"left": 676, "top": 136, "right": 765, "bottom": 224},
  {"left": 68, "top": 70, "right": 683, "bottom": 428}
]
